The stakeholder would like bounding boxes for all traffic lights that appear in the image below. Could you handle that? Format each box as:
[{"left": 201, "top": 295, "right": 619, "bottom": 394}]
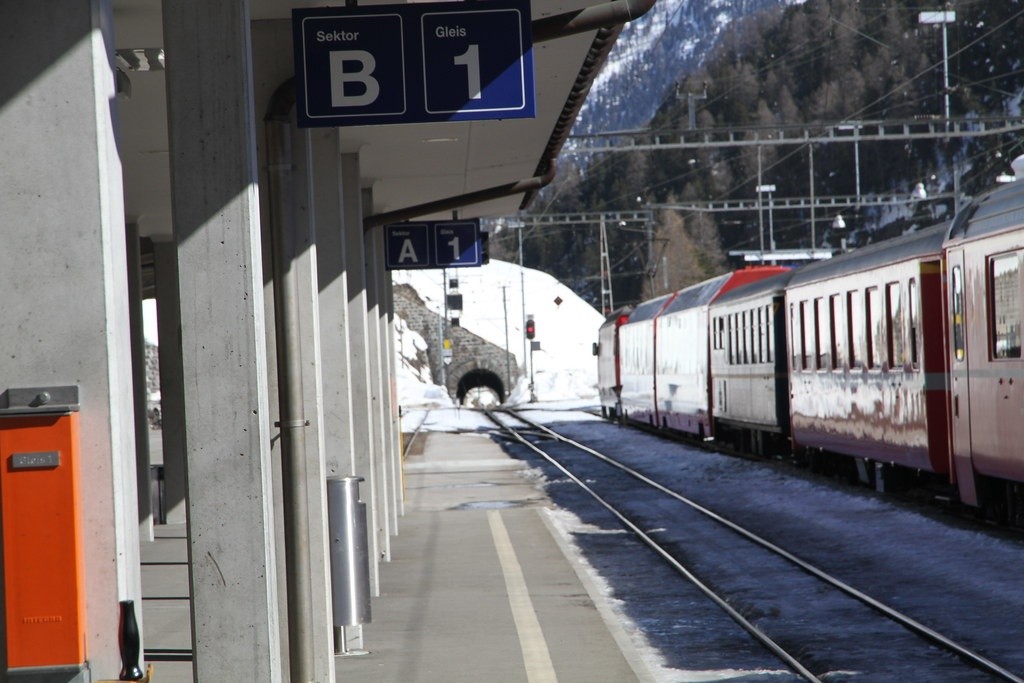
[{"left": 527, "top": 320, "right": 536, "bottom": 338}]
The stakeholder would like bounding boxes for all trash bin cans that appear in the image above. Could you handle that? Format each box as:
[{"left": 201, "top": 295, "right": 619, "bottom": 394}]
[{"left": 327, "top": 474, "right": 372, "bottom": 656}]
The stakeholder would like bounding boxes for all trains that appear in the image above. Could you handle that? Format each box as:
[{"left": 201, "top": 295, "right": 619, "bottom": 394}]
[{"left": 589, "top": 173, "right": 1024, "bottom": 522}]
